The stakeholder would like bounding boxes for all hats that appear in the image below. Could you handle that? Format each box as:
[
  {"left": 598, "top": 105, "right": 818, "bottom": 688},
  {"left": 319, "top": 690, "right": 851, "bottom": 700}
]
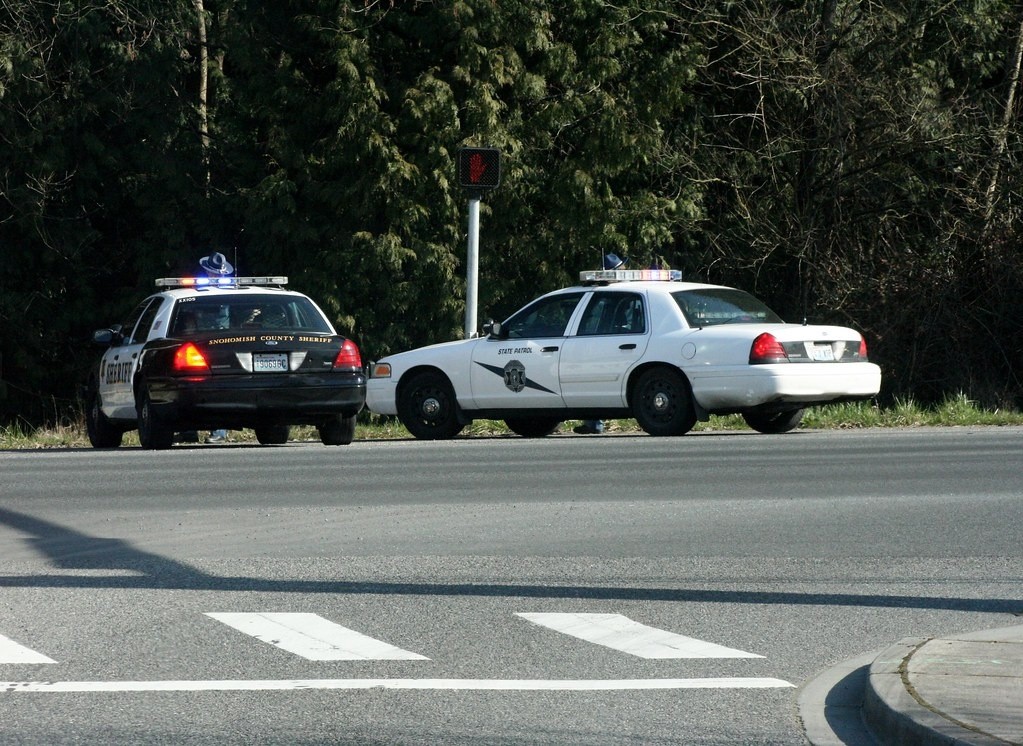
[
  {"left": 200, "top": 252, "right": 234, "bottom": 274},
  {"left": 596, "top": 253, "right": 629, "bottom": 271}
]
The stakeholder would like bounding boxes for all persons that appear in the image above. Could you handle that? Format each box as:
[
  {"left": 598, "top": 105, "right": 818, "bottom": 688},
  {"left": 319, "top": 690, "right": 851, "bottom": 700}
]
[
  {"left": 572, "top": 252, "right": 637, "bottom": 433},
  {"left": 171, "top": 251, "right": 236, "bottom": 446}
]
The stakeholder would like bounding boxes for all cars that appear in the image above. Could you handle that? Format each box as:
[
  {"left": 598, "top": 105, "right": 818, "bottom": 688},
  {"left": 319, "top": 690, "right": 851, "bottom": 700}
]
[
  {"left": 364, "top": 269, "right": 881, "bottom": 442},
  {"left": 86, "top": 277, "right": 366, "bottom": 449}
]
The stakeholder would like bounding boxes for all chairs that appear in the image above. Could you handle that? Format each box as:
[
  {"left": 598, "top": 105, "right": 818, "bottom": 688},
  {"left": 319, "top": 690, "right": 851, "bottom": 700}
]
[{"left": 613, "top": 310, "right": 630, "bottom": 333}]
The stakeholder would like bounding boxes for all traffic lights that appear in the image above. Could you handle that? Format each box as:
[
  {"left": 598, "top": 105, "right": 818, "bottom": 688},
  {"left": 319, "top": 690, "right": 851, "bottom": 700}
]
[{"left": 459, "top": 146, "right": 502, "bottom": 189}]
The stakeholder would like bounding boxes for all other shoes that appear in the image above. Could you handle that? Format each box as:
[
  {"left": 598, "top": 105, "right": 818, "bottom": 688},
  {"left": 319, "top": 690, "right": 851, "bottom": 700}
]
[
  {"left": 204, "top": 436, "right": 224, "bottom": 444},
  {"left": 572, "top": 425, "right": 602, "bottom": 435},
  {"left": 174, "top": 434, "right": 199, "bottom": 443}
]
